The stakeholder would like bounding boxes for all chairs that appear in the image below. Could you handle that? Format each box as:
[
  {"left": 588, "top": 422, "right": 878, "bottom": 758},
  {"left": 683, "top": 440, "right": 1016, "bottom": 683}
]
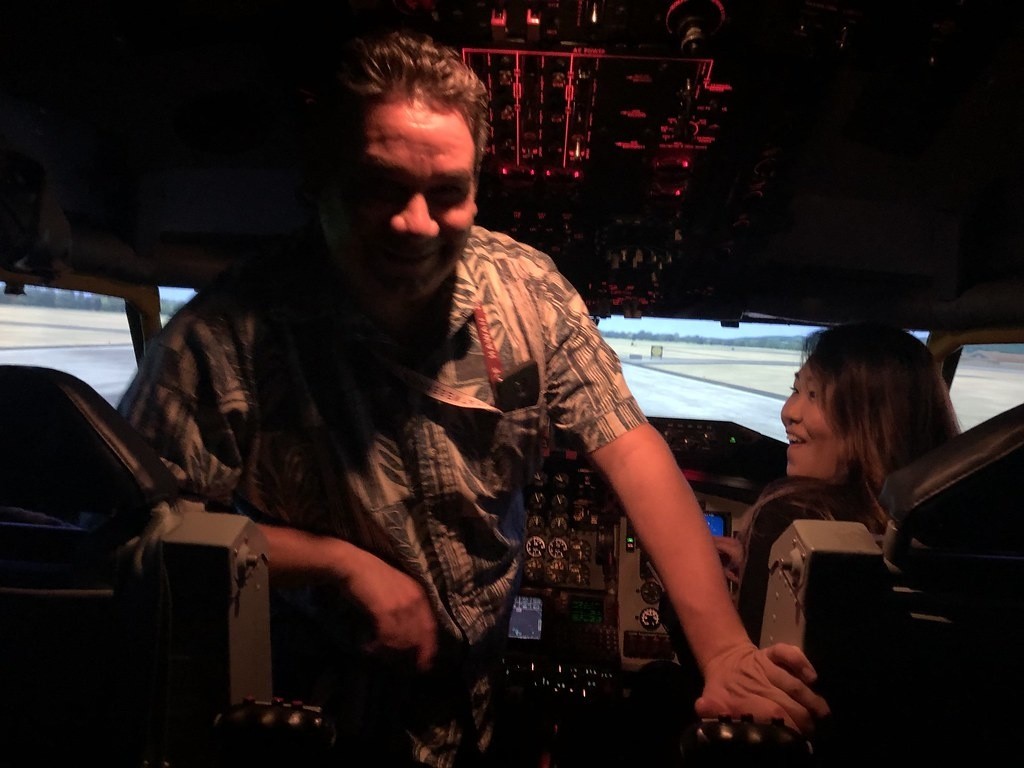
[
  {"left": 682, "top": 405, "right": 1024, "bottom": 768},
  {"left": 0, "top": 365, "right": 337, "bottom": 768}
]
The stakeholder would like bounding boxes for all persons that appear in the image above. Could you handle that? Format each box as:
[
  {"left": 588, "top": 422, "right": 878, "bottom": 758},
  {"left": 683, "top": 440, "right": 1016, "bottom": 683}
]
[
  {"left": 116, "top": 34, "right": 830, "bottom": 738},
  {"left": 709, "top": 325, "right": 963, "bottom": 648}
]
